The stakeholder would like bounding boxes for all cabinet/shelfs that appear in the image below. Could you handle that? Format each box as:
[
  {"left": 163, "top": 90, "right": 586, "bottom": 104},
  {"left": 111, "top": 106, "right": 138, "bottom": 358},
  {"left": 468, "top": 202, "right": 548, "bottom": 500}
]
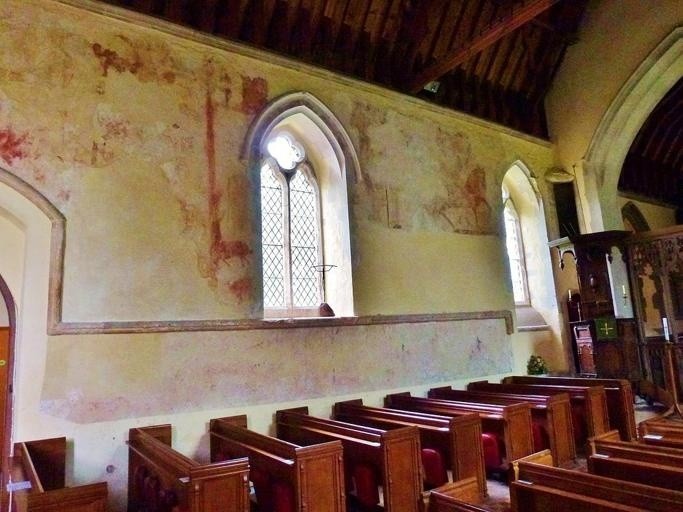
[{"left": 545, "top": 228, "right": 649, "bottom": 398}]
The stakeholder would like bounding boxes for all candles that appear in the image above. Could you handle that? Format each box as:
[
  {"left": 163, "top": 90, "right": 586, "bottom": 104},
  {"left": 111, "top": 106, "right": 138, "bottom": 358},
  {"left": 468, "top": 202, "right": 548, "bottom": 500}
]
[
  {"left": 568, "top": 289, "right": 572, "bottom": 298},
  {"left": 622, "top": 285, "right": 625, "bottom": 294}
]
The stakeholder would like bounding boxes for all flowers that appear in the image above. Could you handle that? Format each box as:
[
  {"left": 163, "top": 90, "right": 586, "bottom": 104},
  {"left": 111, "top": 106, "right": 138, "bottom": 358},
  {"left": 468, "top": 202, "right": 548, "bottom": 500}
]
[{"left": 527, "top": 355, "right": 549, "bottom": 375}]
